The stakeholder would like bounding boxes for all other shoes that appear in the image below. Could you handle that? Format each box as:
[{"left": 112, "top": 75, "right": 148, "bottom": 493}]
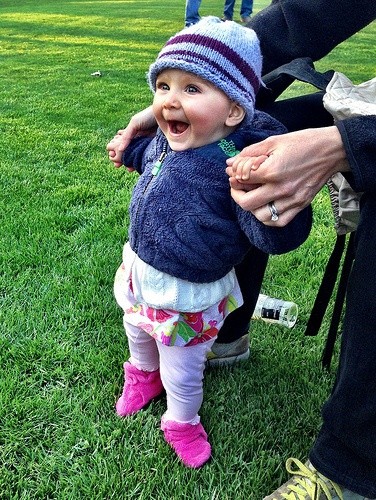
[
  {"left": 221, "top": 15, "right": 233, "bottom": 21},
  {"left": 162, "top": 415, "right": 211, "bottom": 468},
  {"left": 240, "top": 16, "right": 252, "bottom": 23},
  {"left": 116, "top": 362, "right": 162, "bottom": 416}
]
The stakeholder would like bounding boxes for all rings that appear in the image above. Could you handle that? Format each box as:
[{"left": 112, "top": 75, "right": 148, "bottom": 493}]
[{"left": 268, "top": 203, "right": 279, "bottom": 222}]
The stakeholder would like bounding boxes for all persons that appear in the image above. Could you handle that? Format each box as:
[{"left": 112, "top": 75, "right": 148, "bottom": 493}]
[
  {"left": 184, "top": 0, "right": 202, "bottom": 28},
  {"left": 106, "top": 0, "right": 376, "bottom": 500},
  {"left": 106, "top": 16, "right": 312, "bottom": 468},
  {"left": 221, "top": 0, "right": 254, "bottom": 23}
]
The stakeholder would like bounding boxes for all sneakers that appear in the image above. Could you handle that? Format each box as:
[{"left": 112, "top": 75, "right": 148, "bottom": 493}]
[
  {"left": 205, "top": 333, "right": 250, "bottom": 365},
  {"left": 262, "top": 459, "right": 370, "bottom": 500}
]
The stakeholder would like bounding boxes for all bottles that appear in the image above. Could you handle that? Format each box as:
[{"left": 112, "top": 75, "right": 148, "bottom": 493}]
[{"left": 251, "top": 293, "right": 299, "bottom": 329}]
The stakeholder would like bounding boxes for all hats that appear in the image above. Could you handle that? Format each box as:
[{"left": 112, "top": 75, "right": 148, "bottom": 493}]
[{"left": 147, "top": 15, "right": 262, "bottom": 125}]
